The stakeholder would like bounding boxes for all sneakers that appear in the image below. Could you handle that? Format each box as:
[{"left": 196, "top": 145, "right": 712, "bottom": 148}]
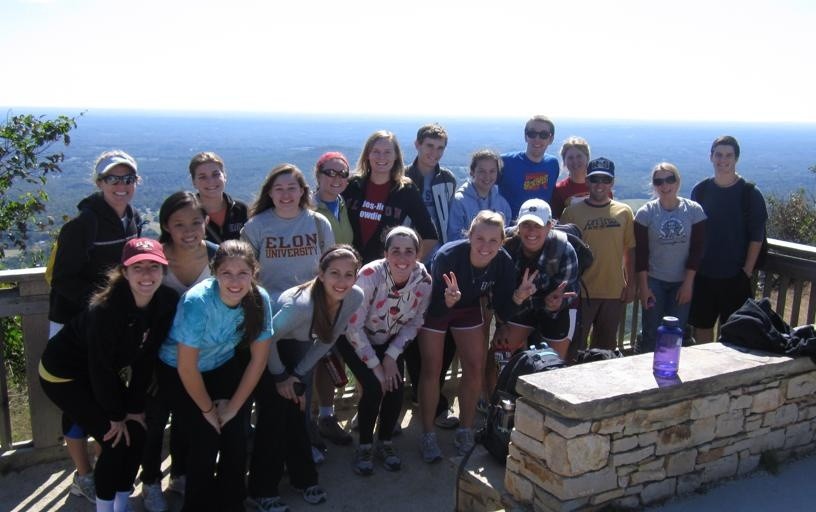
[
  {"left": 246, "top": 495, "right": 293, "bottom": 512},
  {"left": 142, "top": 482, "right": 168, "bottom": 512},
  {"left": 290, "top": 484, "right": 327, "bottom": 505},
  {"left": 70, "top": 469, "right": 96, "bottom": 503},
  {"left": 421, "top": 431, "right": 443, "bottom": 463},
  {"left": 169, "top": 477, "right": 184, "bottom": 493},
  {"left": 376, "top": 444, "right": 401, "bottom": 471},
  {"left": 354, "top": 447, "right": 373, "bottom": 474},
  {"left": 454, "top": 428, "right": 475, "bottom": 455},
  {"left": 317, "top": 414, "right": 353, "bottom": 445}
]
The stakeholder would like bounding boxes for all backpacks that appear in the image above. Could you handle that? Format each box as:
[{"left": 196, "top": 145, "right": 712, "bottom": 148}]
[
  {"left": 504, "top": 223, "right": 594, "bottom": 310},
  {"left": 485, "top": 341, "right": 565, "bottom": 465}
]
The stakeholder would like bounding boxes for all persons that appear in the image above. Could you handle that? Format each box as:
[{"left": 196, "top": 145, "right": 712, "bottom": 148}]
[
  {"left": 554, "top": 135, "right": 769, "bottom": 363},
  {"left": 40, "top": 114, "right": 577, "bottom": 511}
]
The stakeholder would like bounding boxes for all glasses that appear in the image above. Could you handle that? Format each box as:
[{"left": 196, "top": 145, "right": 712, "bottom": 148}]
[
  {"left": 528, "top": 129, "right": 551, "bottom": 140},
  {"left": 104, "top": 172, "right": 136, "bottom": 185},
  {"left": 653, "top": 175, "right": 675, "bottom": 186},
  {"left": 589, "top": 175, "right": 612, "bottom": 185},
  {"left": 321, "top": 166, "right": 350, "bottom": 179}
]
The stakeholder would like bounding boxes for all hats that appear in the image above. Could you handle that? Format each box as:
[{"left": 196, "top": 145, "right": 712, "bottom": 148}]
[
  {"left": 517, "top": 198, "right": 552, "bottom": 225},
  {"left": 316, "top": 152, "right": 350, "bottom": 172},
  {"left": 587, "top": 157, "right": 614, "bottom": 179},
  {"left": 123, "top": 238, "right": 169, "bottom": 267},
  {"left": 95, "top": 151, "right": 137, "bottom": 180}
]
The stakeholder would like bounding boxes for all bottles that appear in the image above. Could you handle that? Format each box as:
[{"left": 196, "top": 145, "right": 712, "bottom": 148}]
[
  {"left": 652, "top": 315, "right": 686, "bottom": 380},
  {"left": 322, "top": 349, "right": 348, "bottom": 389}
]
